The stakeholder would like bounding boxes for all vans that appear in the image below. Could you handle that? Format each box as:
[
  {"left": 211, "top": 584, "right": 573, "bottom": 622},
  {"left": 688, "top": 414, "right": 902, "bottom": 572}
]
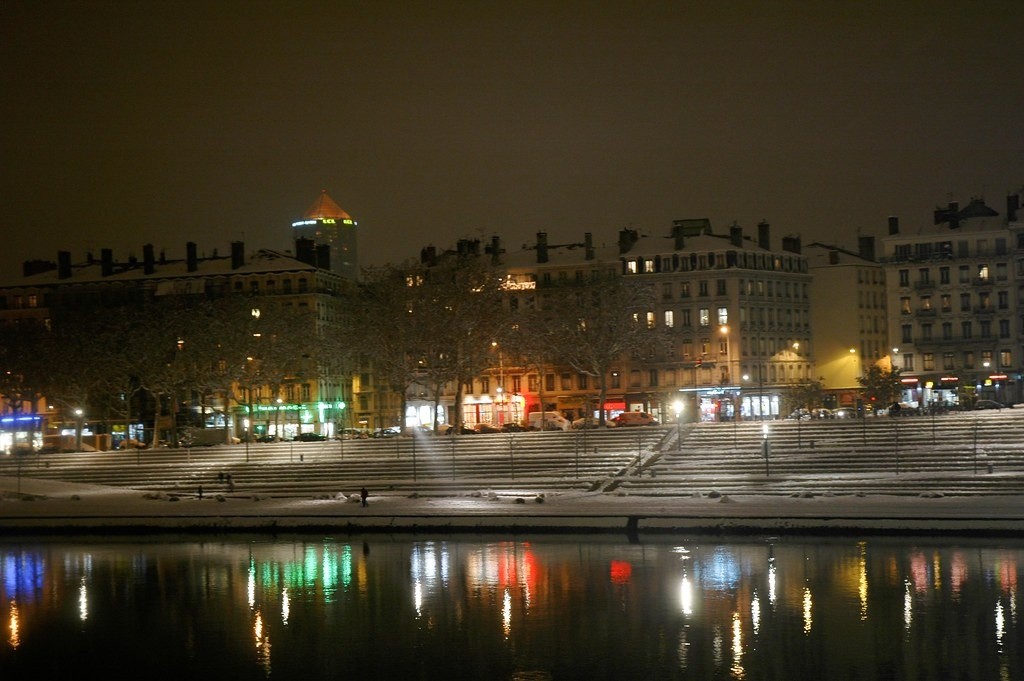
[
  {"left": 405, "top": 426, "right": 421, "bottom": 437},
  {"left": 619, "top": 412, "right": 656, "bottom": 427},
  {"left": 264, "top": 434, "right": 275, "bottom": 443},
  {"left": 930, "top": 400, "right": 960, "bottom": 415},
  {"left": 529, "top": 412, "right": 569, "bottom": 431}
]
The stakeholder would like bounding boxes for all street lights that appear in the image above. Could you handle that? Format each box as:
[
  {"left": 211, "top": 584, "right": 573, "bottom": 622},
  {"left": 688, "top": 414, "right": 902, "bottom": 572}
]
[
  {"left": 277, "top": 399, "right": 301, "bottom": 435},
  {"left": 763, "top": 426, "right": 769, "bottom": 477},
  {"left": 491, "top": 337, "right": 504, "bottom": 423},
  {"left": 675, "top": 402, "right": 681, "bottom": 451},
  {"left": 721, "top": 325, "right": 733, "bottom": 420}
]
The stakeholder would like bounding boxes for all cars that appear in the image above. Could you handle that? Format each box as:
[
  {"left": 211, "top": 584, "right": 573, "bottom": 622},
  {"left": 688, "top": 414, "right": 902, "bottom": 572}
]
[
  {"left": 119, "top": 439, "right": 146, "bottom": 450},
  {"left": 572, "top": 418, "right": 616, "bottom": 428},
  {"left": 474, "top": 423, "right": 501, "bottom": 433},
  {"left": 335, "top": 429, "right": 361, "bottom": 440},
  {"left": 446, "top": 426, "right": 478, "bottom": 433},
  {"left": 293, "top": 433, "right": 326, "bottom": 441},
  {"left": 790, "top": 407, "right": 856, "bottom": 420},
  {"left": 374, "top": 429, "right": 399, "bottom": 438},
  {"left": 974, "top": 400, "right": 1004, "bottom": 409},
  {"left": 148, "top": 440, "right": 171, "bottom": 448},
  {"left": 502, "top": 424, "right": 527, "bottom": 432}
]
